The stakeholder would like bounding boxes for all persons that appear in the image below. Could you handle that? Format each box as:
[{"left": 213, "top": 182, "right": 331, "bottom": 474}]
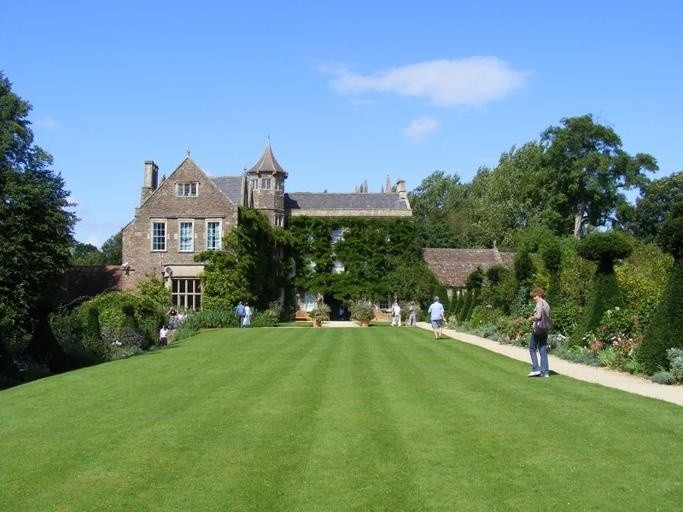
[
  {"left": 389, "top": 303, "right": 401, "bottom": 328},
  {"left": 526, "top": 287, "right": 551, "bottom": 377},
  {"left": 234, "top": 300, "right": 245, "bottom": 328},
  {"left": 406, "top": 301, "right": 416, "bottom": 326},
  {"left": 165, "top": 306, "right": 178, "bottom": 329},
  {"left": 242, "top": 302, "right": 252, "bottom": 328},
  {"left": 158, "top": 324, "right": 169, "bottom": 346},
  {"left": 427, "top": 295, "right": 446, "bottom": 340}
]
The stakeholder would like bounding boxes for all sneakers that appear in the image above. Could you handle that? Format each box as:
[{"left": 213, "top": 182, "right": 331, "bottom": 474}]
[
  {"left": 527, "top": 370, "right": 540, "bottom": 377},
  {"left": 540, "top": 373, "right": 549, "bottom": 378}
]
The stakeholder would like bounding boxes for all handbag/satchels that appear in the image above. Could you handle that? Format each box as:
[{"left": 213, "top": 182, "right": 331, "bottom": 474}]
[{"left": 534, "top": 300, "right": 550, "bottom": 336}]
